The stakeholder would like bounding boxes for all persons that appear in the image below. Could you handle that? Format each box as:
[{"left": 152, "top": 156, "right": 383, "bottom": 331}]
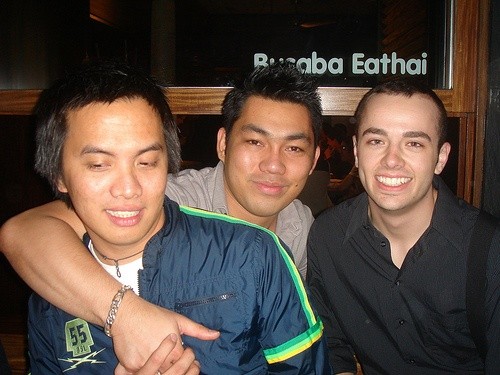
[
  {"left": 26, "top": 69, "right": 332, "bottom": 375},
  {"left": 304, "top": 123, "right": 364, "bottom": 221},
  {"left": 0, "top": 60, "right": 325, "bottom": 375},
  {"left": 305, "top": 79, "right": 500, "bottom": 375}
]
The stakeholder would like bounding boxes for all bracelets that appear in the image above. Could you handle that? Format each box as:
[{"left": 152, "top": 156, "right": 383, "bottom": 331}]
[{"left": 104, "top": 285, "right": 134, "bottom": 337}]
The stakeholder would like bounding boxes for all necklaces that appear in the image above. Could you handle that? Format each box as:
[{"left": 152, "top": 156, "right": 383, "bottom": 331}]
[{"left": 94, "top": 245, "right": 143, "bottom": 278}]
